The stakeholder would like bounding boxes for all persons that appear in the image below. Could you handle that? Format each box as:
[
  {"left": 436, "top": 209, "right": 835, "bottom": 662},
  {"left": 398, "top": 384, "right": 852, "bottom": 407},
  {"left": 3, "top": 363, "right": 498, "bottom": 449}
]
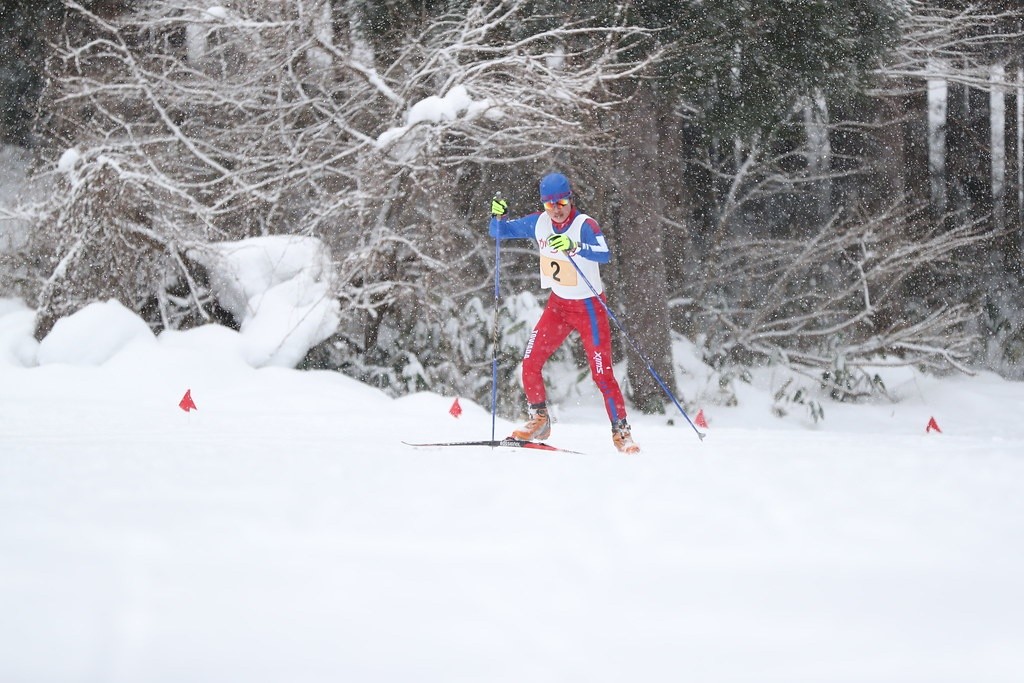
[{"left": 488, "top": 174, "right": 640, "bottom": 452}]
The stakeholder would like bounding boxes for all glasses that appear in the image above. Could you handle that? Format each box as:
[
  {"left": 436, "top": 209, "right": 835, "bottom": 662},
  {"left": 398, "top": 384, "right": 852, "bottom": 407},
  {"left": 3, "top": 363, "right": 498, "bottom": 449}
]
[{"left": 543, "top": 196, "right": 569, "bottom": 210}]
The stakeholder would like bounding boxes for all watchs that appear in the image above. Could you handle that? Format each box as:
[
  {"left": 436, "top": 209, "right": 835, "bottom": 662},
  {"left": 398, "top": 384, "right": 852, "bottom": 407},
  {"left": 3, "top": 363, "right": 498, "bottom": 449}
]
[{"left": 574, "top": 242, "right": 582, "bottom": 253}]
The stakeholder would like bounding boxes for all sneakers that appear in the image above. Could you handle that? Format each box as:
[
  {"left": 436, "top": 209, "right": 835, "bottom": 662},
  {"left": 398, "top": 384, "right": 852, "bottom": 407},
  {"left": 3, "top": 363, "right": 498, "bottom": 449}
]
[
  {"left": 612, "top": 420, "right": 639, "bottom": 453},
  {"left": 514, "top": 406, "right": 551, "bottom": 440}
]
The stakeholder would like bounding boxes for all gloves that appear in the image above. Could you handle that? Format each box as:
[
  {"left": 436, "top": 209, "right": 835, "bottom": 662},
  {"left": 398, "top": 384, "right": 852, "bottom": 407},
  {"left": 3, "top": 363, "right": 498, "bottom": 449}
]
[
  {"left": 547, "top": 234, "right": 576, "bottom": 253},
  {"left": 491, "top": 193, "right": 508, "bottom": 217}
]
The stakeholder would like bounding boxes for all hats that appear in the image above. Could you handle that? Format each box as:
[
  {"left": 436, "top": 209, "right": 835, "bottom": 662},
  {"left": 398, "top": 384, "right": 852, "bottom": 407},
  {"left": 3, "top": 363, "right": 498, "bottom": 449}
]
[{"left": 538, "top": 173, "right": 572, "bottom": 203}]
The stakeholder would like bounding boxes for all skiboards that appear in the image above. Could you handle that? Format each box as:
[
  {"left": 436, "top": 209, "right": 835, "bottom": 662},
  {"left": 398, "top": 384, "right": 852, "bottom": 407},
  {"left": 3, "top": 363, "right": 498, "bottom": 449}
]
[{"left": 401, "top": 440, "right": 586, "bottom": 457}]
